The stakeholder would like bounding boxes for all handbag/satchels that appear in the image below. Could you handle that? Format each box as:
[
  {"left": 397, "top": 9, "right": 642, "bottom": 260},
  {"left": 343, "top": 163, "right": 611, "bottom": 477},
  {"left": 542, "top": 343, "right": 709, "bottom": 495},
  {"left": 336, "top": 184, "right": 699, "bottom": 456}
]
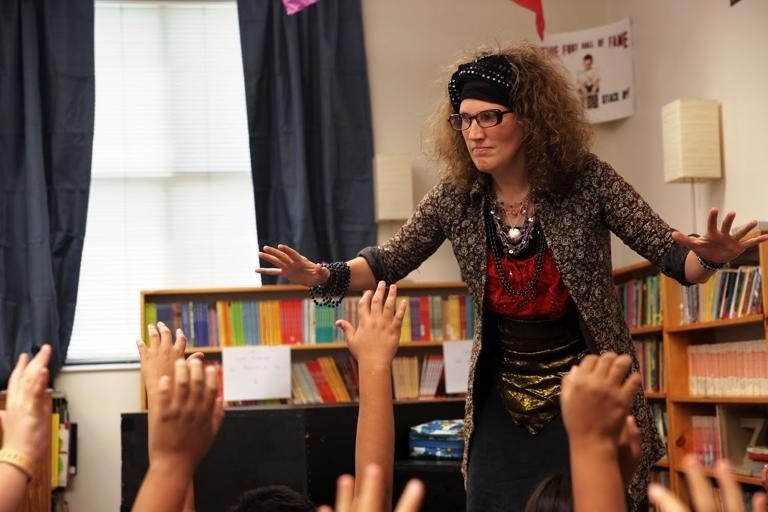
[{"left": 408, "top": 419, "right": 465, "bottom": 460}]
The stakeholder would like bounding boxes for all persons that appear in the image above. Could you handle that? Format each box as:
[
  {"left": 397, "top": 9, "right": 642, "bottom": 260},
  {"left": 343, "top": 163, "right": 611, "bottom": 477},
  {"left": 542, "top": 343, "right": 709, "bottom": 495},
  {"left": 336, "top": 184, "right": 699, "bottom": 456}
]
[
  {"left": 126, "top": 320, "right": 225, "bottom": 510},
  {"left": 1, "top": 342, "right": 57, "bottom": 511},
  {"left": 575, "top": 54, "right": 599, "bottom": 97},
  {"left": 254, "top": 43, "right": 768, "bottom": 511},
  {"left": 558, "top": 351, "right": 766, "bottom": 510},
  {"left": 323, "top": 280, "right": 425, "bottom": 511}
]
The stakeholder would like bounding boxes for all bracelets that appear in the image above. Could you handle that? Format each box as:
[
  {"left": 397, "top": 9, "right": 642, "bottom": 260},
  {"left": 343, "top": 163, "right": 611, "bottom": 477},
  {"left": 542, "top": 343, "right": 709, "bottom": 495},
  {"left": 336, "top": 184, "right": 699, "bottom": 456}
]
[
  {"left": 309, "top": 261, "right": 351, "bottom": 309},
  {"left": 695, "top": 256, "right": 726, "bottom": 272}
]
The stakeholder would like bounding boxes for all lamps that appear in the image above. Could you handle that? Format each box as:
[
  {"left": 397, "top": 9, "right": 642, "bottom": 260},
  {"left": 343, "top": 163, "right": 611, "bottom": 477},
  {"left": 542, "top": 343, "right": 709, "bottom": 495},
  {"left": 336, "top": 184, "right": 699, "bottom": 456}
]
[{"left": 660, "top": 98, "right": 725, "bottom": 244}]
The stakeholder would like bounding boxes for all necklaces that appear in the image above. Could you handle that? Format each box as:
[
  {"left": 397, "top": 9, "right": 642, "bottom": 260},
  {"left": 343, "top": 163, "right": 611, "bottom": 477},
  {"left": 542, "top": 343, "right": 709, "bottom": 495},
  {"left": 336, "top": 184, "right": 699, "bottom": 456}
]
[{"left": 484, "top": 189, "right": 547, "bottom": 306}]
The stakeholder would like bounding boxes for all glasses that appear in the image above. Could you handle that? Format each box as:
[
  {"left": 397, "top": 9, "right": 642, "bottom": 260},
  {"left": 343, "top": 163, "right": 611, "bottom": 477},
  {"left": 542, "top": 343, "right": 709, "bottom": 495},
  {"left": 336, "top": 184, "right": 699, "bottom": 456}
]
[{"left": 447, "top": 109, "right": 512, "bottom": 131}]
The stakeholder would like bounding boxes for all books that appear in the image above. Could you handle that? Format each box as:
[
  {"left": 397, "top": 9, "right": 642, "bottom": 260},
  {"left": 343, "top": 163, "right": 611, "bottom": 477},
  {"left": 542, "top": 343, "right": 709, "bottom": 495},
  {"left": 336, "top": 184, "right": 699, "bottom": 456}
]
[
  {"left": 612, "top": 266, "right": 768, "bottom": 511},
  {"left": 144, "top": 294, "right": 478, "bottom": 406},
  {"left": 1, "top": 388, "right": 81, "bottom": 486}
]
